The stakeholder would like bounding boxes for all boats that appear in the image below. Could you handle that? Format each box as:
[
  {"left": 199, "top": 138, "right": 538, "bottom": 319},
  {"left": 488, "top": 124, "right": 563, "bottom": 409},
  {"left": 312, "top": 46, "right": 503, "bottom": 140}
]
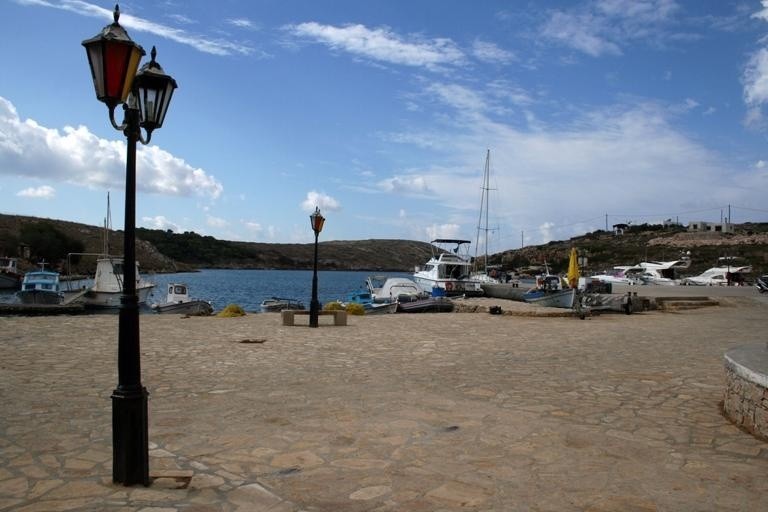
[
  {"left": 260, "top": 297, "right": 306, "bottom": 313},
  {"left": 0, "top": 191, "right": 215, "bottom": 315},
  {"left": 343, "top": 149, "right": 576, "bottom": 312}
]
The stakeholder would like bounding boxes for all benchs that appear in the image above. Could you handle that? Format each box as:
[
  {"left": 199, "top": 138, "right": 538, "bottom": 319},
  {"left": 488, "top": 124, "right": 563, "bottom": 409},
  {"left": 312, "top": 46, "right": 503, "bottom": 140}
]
[{"left": 283, "top": 308, "right": 348, "bottom": 328}]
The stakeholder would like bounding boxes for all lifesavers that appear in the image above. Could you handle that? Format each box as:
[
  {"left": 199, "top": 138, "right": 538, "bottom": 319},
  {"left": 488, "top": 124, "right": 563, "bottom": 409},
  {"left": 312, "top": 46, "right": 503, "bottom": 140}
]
[{"left": 445, "top": 282, "right": 453, "bottom": 289}]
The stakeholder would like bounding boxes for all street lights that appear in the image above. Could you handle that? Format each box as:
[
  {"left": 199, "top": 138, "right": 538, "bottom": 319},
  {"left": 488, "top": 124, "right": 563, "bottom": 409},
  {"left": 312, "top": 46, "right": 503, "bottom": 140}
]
[
  {"left": 81, "top": 4, "right": 178, "bottom": 488},
  {"left": 309, "top": 206, "right": 325, "bottom": 327}
]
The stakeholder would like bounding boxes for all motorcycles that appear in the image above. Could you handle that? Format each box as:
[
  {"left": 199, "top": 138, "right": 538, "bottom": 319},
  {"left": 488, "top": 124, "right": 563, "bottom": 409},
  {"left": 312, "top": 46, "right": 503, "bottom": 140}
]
[{"left": 753, "top": 276, "right": 768, "bottom": 293}]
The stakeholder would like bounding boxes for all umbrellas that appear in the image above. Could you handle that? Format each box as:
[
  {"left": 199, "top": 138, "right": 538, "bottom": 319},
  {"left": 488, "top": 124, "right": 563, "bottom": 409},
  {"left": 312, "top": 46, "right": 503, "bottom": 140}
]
[{"left": 568, "top": 248, "right": 580, "bottom": 288}]
